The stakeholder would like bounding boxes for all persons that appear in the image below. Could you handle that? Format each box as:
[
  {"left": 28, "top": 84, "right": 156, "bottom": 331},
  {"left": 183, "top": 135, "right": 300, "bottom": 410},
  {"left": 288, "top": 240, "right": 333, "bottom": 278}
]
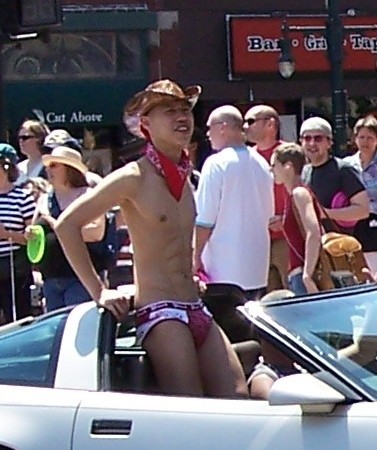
[
  {"left": 248, "top": 289, "right": 377, "bottom": 401},
  {"left": 32, "top": 146, "right": 109, "bottom": 311},
  {"left": 55, "top": 80, "right": 250, "bottom": 399},
  {"left": 43, "top": 130, "right": 103, "bottom": 188},
  {"left": 0, "top": 143, "right": 37, "bottom": 325},
  {"left": 343, "top": 116, "right": 377, "bottom": 254},
  {"left": 243, "top": 104, "right": 285, "bottom": 291},
  {"left": 299, "top": 117, "right": 371, "bottom": 235},
  {"left": 17, "top": 120, "right": 51, "bottom": 179},
  {"left": 192, "top": 105, "right": 271, "bottom": 302},
  {"left": 270, "top": 142, "right": 322, "bottom": 294}
]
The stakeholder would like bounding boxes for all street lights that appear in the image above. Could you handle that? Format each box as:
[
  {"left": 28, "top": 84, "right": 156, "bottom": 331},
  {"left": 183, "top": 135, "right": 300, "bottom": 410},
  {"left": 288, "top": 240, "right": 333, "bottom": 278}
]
[{"left": 276, "top": 5, "right": 350, "bottom": 157}]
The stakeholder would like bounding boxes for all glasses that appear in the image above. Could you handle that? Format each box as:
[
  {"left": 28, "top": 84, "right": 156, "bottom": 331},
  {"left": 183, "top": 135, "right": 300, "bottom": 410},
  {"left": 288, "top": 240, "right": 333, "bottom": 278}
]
[
  {"left": 303, "top": 135, "right": 329, "bottom": 142},
  {"left": 244, "top": 117, "right": 270, "bottom": 125},
  {"left": 18, "top": 134, "right": 36, "bottom": 140}
]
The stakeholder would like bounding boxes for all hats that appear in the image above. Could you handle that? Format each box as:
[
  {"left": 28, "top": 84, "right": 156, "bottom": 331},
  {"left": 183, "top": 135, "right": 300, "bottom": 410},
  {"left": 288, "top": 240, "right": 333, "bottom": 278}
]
[
  {"left": 41, "top": 148, "right": 88, "bottom": 177},
  {"left": 0, "top": 143, "right": 17, "bottom": 164},
  {"left": 123, "top": 80, "right": 202, "bottom": 140},
  {"left": 43, "top": 129, "right": 82, "bottom": 149}
]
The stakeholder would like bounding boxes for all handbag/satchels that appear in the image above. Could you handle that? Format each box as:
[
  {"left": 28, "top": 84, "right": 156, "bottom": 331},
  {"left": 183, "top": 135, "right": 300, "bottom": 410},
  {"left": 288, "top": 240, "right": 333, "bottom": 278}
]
[
  {"left": 90, "top": 211, "right": 124, "bottom": 272},
  {"left": 318, "top": 232, "right": 373, "bottom": 290}
]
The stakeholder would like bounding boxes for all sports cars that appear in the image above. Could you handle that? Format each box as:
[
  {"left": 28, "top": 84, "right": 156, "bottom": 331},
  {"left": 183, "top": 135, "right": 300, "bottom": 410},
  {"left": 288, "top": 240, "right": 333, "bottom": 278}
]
[{"left": 0, "top": 285, "right": 377, "bottom": 450}]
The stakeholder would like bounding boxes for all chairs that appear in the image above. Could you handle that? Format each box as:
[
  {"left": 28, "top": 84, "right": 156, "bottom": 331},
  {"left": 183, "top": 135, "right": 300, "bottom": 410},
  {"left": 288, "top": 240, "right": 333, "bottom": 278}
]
[
  {"left": 231, "top": 339, "right": 262, "bottom": 375},
  {"left": 126, "top": 356, "right": 151, "bottom": 391}
]
